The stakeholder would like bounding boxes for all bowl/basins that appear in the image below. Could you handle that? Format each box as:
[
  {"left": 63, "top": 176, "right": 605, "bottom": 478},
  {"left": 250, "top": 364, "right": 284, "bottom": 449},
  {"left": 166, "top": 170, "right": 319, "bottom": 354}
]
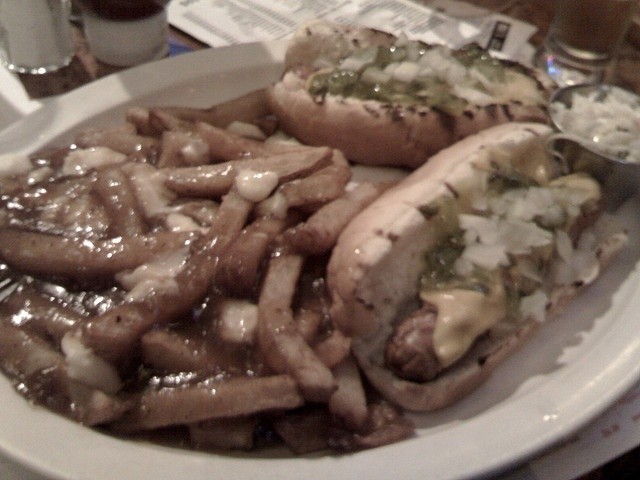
[{"left": 545, "top": 81, "right": 639, "bottom": 213}]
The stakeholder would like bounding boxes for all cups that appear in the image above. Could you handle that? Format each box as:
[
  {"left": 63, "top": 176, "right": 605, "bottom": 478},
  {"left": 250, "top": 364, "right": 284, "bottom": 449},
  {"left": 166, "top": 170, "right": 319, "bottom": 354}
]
[
  {"left": 1, "top": 2, "right": 75, "bottom": 74},
  {"left": 80, "top": 1, "right": 170, "bottom": 66},
  {"left": 531, "top": 0, "right": 638, "bottom": 88}
]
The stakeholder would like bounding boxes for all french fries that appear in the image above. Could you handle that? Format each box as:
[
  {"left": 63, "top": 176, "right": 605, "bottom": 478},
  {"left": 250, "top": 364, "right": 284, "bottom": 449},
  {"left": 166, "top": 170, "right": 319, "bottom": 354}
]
[{"left": 1, "top": 89, "right": 414, "bottom": 456}]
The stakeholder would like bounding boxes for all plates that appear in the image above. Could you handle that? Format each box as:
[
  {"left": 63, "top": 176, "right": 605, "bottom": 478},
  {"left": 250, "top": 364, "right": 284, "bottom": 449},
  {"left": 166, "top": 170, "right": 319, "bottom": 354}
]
[{"left": 0, "top": 38, "right": 639, "bottom": 478}]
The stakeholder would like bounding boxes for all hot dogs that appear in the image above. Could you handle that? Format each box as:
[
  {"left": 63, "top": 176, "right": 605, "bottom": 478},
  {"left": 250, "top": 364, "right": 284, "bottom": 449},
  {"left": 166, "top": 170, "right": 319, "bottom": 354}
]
[
  {"left": 325, "top": 121, "right": 628, "bottom": 413},
  {"left": 266, "top": 23, "right": 546, "bottom": 165}
]
